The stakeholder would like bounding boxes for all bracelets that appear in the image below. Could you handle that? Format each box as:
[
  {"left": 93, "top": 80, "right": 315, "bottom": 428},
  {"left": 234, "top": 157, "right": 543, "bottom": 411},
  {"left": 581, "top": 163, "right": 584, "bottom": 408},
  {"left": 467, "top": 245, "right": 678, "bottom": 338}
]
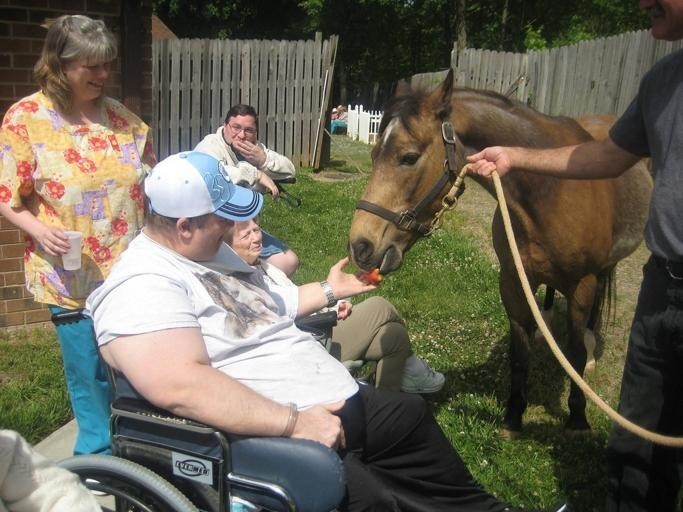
[
  {"left": 321, "top": 279, "right": 337, "bottom": 308},
  {"left": 281, "top": 401, "right": 299, "bottom": 437}
]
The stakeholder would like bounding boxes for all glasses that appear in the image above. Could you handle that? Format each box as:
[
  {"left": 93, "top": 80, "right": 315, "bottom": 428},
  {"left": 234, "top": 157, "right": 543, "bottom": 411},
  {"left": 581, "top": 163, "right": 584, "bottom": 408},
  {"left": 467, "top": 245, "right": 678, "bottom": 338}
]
[{"left": 228, "top": 122, "right": 257, "bottom": 137}]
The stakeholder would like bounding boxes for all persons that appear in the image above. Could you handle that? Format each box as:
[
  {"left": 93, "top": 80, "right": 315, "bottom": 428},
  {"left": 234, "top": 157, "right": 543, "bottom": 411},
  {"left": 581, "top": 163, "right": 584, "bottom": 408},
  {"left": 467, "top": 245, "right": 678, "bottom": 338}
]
[
  {"left": 231, "top": 218, "right": 448, "bottom": 396},
  {"left": 1, "top": 13, "right": 159, "bottom": 459},
  {"left": 331, "top": 105, "right": 349, "bottom": 135},
  {"left": 331, "top": 107, "right": 339, "bottom": 120},
  {"left": 1, "top": 429, "right": 104, "bottom": 511},
  {"left": 468, "top": 0, "right": 682, "bottom": 509},
  {"left": 85, "top": 148, "right": 566, "bottom": 510},
  {"left": 192, "top": 104, "right": 301, "bottom": 280}
]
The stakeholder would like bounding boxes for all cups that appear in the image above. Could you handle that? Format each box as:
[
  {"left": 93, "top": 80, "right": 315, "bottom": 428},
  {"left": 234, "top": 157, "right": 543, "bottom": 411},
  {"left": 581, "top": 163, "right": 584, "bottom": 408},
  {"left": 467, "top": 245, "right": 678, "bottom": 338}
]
[{"left": 61, "top": 232, "right": 82, "bottom": 270}]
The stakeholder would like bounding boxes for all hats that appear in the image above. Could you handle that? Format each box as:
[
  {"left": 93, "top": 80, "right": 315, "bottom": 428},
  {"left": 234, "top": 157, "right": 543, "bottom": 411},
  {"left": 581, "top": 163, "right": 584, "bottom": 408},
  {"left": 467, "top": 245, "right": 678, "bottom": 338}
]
[{"left": 144, "top": 151, "right": 262, "bottom": 222}]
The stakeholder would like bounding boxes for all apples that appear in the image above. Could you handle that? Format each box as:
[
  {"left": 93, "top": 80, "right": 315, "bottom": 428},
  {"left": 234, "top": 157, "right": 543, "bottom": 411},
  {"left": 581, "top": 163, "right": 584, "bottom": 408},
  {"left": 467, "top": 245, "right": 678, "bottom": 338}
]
[{"left": 363, "top": 268, "right": 384, "bottom": 284}]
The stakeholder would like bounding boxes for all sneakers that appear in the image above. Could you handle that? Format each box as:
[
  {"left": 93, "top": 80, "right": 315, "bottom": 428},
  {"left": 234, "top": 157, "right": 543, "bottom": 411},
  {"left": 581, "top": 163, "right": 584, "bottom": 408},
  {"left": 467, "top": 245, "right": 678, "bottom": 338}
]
[{"left": 399, "top": 360, "right": 444, "bottom": 394}]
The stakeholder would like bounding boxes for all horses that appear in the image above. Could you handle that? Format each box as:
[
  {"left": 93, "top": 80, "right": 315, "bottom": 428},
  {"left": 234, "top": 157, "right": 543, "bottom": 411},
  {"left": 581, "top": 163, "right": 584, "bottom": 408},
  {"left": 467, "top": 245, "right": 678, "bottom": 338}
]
[{"left": 348, "top": 66, "right": 655, "bottom": 443}]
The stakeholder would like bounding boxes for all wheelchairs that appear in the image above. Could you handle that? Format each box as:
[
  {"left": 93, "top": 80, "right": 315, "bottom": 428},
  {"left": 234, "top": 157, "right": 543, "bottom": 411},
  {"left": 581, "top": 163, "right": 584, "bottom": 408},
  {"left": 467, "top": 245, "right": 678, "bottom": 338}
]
[{"left": 43, "top": 305, "right": 346, "bottom": 512}]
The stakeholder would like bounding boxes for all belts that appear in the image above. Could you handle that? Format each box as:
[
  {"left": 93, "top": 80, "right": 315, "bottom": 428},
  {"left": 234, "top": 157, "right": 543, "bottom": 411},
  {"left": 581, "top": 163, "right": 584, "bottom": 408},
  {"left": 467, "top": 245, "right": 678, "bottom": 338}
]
[{"left": 654, "top": 257, "right": 683, "bottom": 280}]
